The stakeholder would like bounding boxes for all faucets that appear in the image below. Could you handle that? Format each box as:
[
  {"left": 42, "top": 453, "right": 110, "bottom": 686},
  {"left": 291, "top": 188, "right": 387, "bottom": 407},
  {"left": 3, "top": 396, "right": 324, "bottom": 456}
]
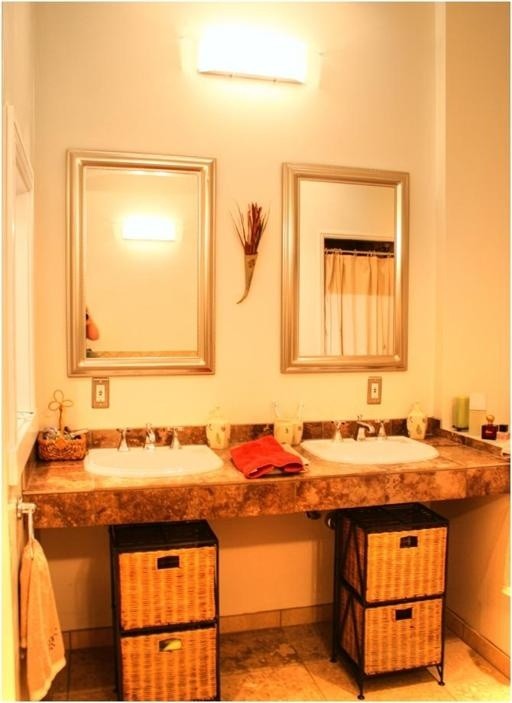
[
  {"left": 145, "top": 424, "right": 156, "bottom": 448},
  {"left": 355, "top": 422, "right": 376, "bottom": 441}
]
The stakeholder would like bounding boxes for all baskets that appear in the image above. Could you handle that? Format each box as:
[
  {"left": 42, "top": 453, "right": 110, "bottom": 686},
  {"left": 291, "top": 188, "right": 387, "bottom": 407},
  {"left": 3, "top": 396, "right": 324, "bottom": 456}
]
[
  {"left": 112, "top": 525, "right": 218, "bottom": 632},
  {"left": 116, "top": 622, "right": 222, "bottom": 700},
  {"left": 336, "top": 581, "right": 446, "bottom": 678},
  {"left": 333, "top": 501, "right": 450, "bottom": 604}
]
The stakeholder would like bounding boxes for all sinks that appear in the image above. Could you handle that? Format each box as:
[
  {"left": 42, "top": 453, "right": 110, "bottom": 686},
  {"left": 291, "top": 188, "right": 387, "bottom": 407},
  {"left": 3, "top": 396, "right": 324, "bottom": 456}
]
[
  {"left": 84, "top": 445, "right": 224, "bottom": 478},
  {"left": 302, "top": 435, "right": 440, "bottom": 465}
]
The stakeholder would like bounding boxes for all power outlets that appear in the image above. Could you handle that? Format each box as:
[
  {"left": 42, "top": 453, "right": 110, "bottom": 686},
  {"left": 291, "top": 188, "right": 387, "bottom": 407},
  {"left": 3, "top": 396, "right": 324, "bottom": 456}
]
[
  {"left": 367, "top": 376, "right": 382, "bottom": 404},
  {"left": 92, "top": 377, "right": 110, "bottom": 408}
]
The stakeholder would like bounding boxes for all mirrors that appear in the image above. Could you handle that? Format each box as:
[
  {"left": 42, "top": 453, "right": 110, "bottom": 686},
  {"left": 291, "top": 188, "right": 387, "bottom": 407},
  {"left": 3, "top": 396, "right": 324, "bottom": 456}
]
[
  {"left": 282, "top": 162, "right": 411, "bottom": 372},
  {"left": 66, "top": 150, "right": 216, "bottom": 376}
]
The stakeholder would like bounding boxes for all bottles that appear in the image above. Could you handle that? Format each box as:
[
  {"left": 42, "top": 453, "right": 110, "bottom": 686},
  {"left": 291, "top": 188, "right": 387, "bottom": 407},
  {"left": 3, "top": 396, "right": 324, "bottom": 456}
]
[{"left": 481, "top": 414, "right": 509, "bottom": 449}]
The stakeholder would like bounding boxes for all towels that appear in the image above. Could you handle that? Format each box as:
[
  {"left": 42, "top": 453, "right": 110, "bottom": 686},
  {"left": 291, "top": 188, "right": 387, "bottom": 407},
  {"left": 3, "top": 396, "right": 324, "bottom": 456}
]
[
  {"left": 230, "top": 434, "right": 310, "bottom": 479},
  {"left": 18, "top": 539, "right": 68, "bottom": 700}
]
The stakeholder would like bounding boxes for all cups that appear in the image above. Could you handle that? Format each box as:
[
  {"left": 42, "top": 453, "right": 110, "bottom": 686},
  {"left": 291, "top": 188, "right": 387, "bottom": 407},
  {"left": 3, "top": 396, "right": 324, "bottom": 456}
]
[{"left": 454, "top": 397, "right": 469, "bottom": 430}]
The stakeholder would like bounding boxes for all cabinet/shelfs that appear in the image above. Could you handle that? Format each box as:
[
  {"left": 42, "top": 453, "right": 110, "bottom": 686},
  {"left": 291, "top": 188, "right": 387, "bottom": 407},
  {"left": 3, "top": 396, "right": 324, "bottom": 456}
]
[
  {"left": 329, "top": 505, "right": 449, "bottom": 700},
  {"left": 108, "top": 519, "right": 221, "bottom": 700}
]
